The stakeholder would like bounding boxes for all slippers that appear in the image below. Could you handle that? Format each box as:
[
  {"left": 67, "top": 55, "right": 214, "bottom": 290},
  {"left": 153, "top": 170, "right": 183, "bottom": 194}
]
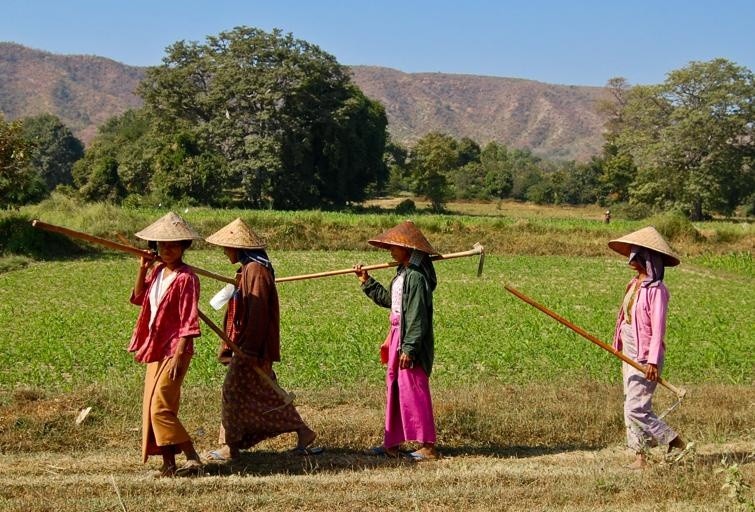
[
  {"left": 362, "top": 447, "right": 385, "bottom": 457},
  {"left": 406, "top": 452, "right": 438, "bottom": 461},
  {"left": 289, "top": 446, "right": 323, "bottom": 456},
  {"left": 660, "top": 441, "right": 695, "bottom": 465},
  {"left": 203, "top": 450, "right": 224, "bottom": 460},
  {"left": 175, "top": 466, "right": 208, "bottom": 476}
]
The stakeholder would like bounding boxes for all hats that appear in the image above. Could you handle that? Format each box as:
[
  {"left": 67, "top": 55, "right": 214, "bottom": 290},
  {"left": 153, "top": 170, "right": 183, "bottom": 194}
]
[
  {"left": 134, "top": 211, "right": 201, "bottom": 242},
  {"left": 204, "top": 217, "right": 268, "bottom": 250},
  {"left": 367, "top": 219, "right": 438, "bottom": 255},
  {"left": 608, "top": 225, "right": 680, "bottom": 267}
]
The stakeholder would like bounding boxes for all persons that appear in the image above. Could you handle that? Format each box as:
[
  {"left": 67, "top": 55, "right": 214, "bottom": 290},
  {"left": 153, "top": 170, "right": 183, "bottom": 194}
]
[
  {"left": 351, "top": 216, "right": 442, "bottom": 462},
  {"left": 127, "top": 209, "right": 205, "bottom": 479},
  {"left": 202, "top": 212, "right": 318, "bottom": 463},
  {"left": 606, "top": 223, "right": 696, "bottom": 471}
]
